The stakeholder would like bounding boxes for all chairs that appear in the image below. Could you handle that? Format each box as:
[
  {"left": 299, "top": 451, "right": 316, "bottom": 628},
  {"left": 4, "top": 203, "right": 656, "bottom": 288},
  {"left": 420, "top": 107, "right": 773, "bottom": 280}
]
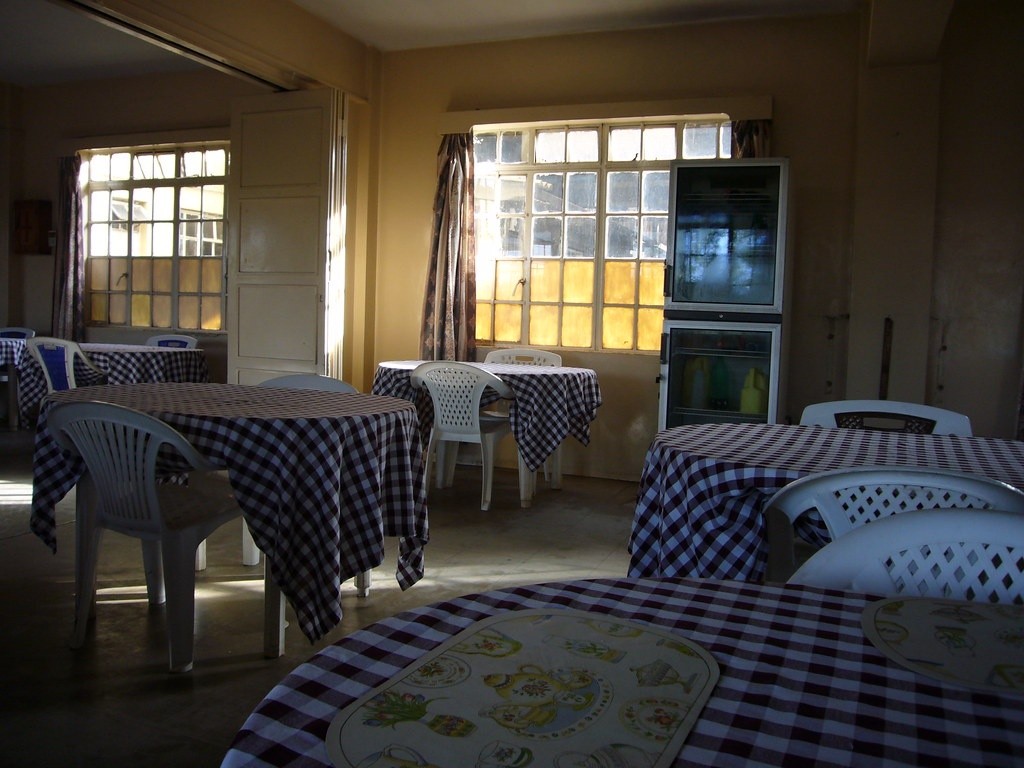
[
  {"left": 27, "top": 337, "right": 110, "bottom": 395},
  {"left": 798, "top": 399, "right": 973, "bottom": 437},
  {"left": 145, "top": 335, "right": 199, "bottom": 349},
  {"left": 410, "top": 361, "right": 512, "bottom": 510},
  {"left": 0, "top": 327, "right": 35, "bottom": 338},
  {"left": 759, "top": 465, "right": 1024, "bottom": 588},
  {"left": 784, "top": 508, "right": 1024, "bottom": 605},
  {"left": 482, "top": 347, "right": 564, "bottom": 489}
]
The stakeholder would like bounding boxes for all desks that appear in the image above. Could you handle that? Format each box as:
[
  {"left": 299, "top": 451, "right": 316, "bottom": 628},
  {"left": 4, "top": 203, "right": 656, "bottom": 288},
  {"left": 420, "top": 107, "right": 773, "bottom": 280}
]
[
  {"left": 30, "top": 382, "right": 431, "bottom": 658},
  {"left": 0, "top": 338, "right": 211, "bottom": 430},
  {"left": 626, "top": 423, "right": 1024, "bottom": 580},
  {"left": 220, "top": 577, "right": 1024, "bottom": 768},
  {"left": 369, "top": 360, "right": 603, "bottom": 508}
]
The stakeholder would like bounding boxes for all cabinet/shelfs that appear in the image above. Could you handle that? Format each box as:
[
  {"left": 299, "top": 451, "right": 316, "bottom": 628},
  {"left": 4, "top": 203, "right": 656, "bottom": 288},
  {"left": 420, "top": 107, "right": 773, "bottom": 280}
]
[{"left": 655, "top": 156, "right": 792, "bottom": 433}]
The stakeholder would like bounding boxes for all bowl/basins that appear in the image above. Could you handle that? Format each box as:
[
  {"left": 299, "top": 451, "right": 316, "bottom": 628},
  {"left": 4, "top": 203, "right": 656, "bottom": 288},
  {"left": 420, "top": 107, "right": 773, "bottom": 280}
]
[
  {"left": 745, "top": 342, "right": 760, "bottom": 351},
  {"left": 711, "top": 399, "right": 727, "bottom": 409}
]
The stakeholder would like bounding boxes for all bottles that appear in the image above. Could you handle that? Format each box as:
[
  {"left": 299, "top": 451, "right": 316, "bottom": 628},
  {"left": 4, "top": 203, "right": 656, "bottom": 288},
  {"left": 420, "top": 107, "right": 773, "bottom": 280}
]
[
  {"left": 740, "top": 367, "right": 766, "bottom": 414},
  {"left": 683, "top": 357, "right": 711, "bottom": 408}
]
